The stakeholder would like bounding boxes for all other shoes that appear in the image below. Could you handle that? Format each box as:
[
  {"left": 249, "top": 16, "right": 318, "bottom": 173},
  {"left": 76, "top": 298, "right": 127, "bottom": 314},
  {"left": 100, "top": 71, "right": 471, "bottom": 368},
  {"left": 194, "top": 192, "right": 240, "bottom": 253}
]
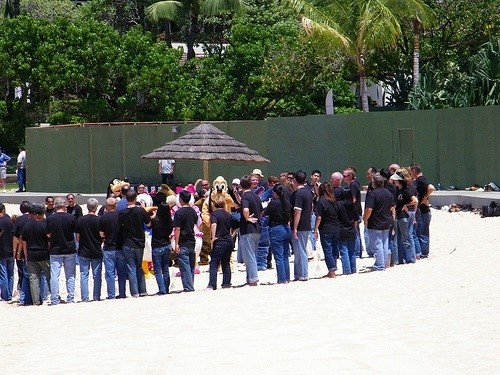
[
  {"left": 241, "top": 282, "right": 257, "bottom": 287},
  {"left": 2, "top": 188, "right": 6, "bottom": 193},
  {"left": 308, "top": 256, "right": 314, "bottom": 262},
  {"left": 16, "top": 188, "right": 23, "bottom": 192},
  {"left": 203, "top": 285, "right": 213, "bottom": 291}
]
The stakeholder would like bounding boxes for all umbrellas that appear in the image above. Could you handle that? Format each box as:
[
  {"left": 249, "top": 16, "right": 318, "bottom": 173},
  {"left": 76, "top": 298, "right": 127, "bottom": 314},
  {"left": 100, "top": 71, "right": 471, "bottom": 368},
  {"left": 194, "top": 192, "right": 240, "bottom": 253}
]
[{"left": 141, "top": 123, "right": 270, "bottom": 180}]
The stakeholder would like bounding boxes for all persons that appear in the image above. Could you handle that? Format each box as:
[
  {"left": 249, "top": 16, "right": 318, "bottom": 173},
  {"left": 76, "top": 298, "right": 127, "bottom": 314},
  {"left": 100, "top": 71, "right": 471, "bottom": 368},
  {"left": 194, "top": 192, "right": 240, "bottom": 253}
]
[
  {"left": 238, "top": 180, "right": 265, "bottom": 286},
  {"left": 364, "top": 161, "right": 436, "bottom": 265},
  {"left": 263, "top": 184, "right": 292, "bottom": 284},
  {"left": 22, "top": 202, "right": 65, "bottom": 305},
  {"left": 227, "top": 166, "right": 363, "bottom": 270},
  {"left": 117, "top": 178, "right": 210, "bottom": 266},
  {"left": 0, "top": 147, "right": 11, "bottom": 193},
  {"left": 362, "top": 175, "right": 397, "bottom": 271},
  {"left": 291, "top": 170, "right": 312, "bottom": 281},
  {"left": 0, "top": 200, "right": 31, "bottom": 306},
  {"left": 158, "top": 158, "right": 175, "bottom": 191},
  {"left": 75, "top": 197, "right": 106, "bottom": 302},
  {"left": 314, "top": 182, "right": 341, "bottom": 278},
  {"left": 46, "top": 197, "right": 77, "bottom": 305},
  {"left": 336, "top": 187, "right": 359, "bottom": 274},
  {"left": 97, "top": 197, "right": 125, "bottom": 298},
  {"left": 43, "top": 194, "right": 109, "bottom": 265},
  {"left": 118, "top": 189, "right": 152, "bottom": 298},
  {"left": 15, "top": 143, "right": 26, "bottom": 192}
]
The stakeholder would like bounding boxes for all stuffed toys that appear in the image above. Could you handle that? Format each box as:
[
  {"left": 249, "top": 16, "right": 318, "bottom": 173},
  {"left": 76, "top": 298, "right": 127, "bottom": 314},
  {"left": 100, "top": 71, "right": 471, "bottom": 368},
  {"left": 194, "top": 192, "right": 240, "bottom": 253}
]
[
  {"left": 169, "top": 184, "right": 205, "bottom": 277},
  {"left": 198, "top": 176, "right": 235, "bottom": 265},
  {"left": 106, "top": 177, "right": 130, "bottom": 198}
]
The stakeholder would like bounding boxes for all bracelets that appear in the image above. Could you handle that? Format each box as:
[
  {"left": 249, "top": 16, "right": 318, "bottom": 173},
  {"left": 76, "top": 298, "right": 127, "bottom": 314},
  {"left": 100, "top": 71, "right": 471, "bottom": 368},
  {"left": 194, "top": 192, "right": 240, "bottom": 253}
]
[{"left": 16, "top": 252, "right": 20, "bottom": 255}]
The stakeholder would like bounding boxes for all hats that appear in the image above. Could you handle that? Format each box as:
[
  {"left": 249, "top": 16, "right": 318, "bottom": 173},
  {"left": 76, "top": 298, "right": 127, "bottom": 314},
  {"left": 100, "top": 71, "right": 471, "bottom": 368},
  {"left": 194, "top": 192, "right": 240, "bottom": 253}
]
[
  {"left": 251, "top": 168, "right": 264, "bottom": 178},
  {"left": 231, "top": 178, "right": 240, "bottom": 185},
  {"left": 390, "top": 171, "right": 406, "bottom": 180}
]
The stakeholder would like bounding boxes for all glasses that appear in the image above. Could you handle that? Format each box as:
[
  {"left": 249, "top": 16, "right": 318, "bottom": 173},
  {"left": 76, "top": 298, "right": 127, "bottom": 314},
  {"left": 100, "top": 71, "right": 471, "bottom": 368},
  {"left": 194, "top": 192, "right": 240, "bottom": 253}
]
[
  {"left": 343, "top": 175, "right": 350, "bottom": 178},
  {"left": 201, "top": 184, "right": 208, "bottom": 187}
]
